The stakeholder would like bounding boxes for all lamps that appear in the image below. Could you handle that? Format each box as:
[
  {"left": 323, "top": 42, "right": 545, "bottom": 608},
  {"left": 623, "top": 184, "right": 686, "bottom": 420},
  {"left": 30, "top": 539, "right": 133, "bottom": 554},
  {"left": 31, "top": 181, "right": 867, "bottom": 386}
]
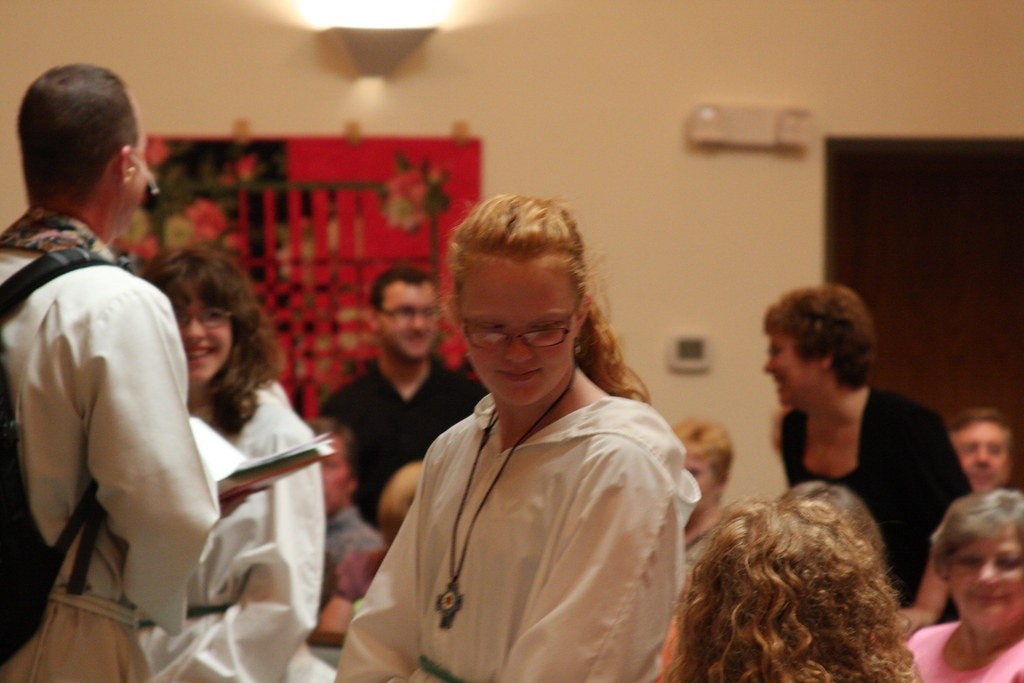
[{"left": 316, "top": 24, "right": 434, "bottom": 72}]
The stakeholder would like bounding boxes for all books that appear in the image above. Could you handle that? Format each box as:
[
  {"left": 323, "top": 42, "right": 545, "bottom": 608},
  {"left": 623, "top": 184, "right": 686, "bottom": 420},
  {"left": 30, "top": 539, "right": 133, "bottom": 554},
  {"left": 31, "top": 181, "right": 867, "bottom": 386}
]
[{"left": 188, "top": 416, "right": 336, "bottom": 501}]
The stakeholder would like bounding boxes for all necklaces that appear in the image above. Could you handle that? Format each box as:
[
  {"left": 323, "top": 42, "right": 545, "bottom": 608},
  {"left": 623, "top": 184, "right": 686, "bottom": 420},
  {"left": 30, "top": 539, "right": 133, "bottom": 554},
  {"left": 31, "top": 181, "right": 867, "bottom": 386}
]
[{"left": 436, "top": 363, "right": 575, "bottom": 628}]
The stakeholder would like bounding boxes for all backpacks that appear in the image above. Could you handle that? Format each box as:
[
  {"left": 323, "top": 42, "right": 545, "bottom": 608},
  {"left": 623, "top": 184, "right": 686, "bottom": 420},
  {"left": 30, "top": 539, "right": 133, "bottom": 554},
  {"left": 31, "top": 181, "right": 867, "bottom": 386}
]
[{"left": 0, "top": 246, "right": 133, "bottom": 668}]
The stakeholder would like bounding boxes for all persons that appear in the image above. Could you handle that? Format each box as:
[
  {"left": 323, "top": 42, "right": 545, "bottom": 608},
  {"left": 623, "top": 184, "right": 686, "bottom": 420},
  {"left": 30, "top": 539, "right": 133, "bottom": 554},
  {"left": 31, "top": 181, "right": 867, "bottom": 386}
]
[
  {"left": 334, "top": 192, "right": 701, "bottom": 682},
  {"left": 1, "top": 64, "right": 1024, "bottom": 683}
]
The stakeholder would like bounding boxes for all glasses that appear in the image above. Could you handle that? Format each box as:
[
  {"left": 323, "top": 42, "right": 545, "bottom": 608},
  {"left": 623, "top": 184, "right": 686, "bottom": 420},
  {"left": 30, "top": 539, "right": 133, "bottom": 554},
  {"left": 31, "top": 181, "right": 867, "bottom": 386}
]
[
  {"left": 178, "top": 308, "right": 230, "bottom": 328},
  {"left": 380, "top": 306, "right": 441, "bottom": 322},
  {"left": 464, "top": 305, "right": 579, "bottom": 349}
]
